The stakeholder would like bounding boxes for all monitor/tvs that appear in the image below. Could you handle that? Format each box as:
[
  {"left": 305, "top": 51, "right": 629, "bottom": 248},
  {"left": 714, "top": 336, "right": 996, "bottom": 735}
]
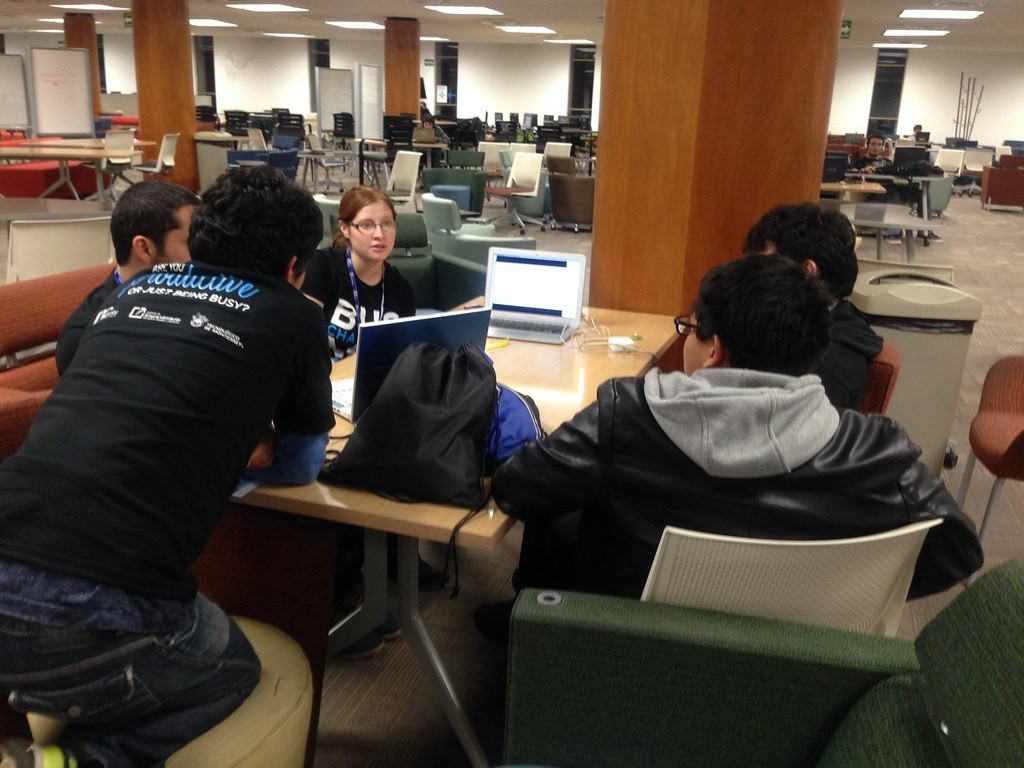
[
  {"left": 510, "top": 113, "right": 521, "bottom": 129},
  {"left": 485, "top": 112, "right": 490, "bottom": 127},
  {"left": 523, "top": 113, "right": 568, "bottom": 130},
  {"left": 495, "top": 113, "right": 503, "bottom": 125}
]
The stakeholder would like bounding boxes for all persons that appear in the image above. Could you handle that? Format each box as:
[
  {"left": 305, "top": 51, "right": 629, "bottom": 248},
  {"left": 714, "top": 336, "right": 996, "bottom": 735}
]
[
  {"left": 54, "top": 181, "right": 402, "bottom": 658},
  {"left": 0, "top": 166, "right": 336, "bottom": 768},
  {"left": 490, "top": 254, "right": 985, "bottom": 600},
  {"left": 848, "top": 134, "right": 943, "bottom": 244},
  {"left": 301, "top": 187, "right": 450, "bottom": 592},
  {"left": 908, "top": 124, "right": 922, "bottom": 139},
  {"left": 742, "top": 202, "right": 885, "bottom": 410},
  {"left": 416, "top": 114, "right": 450, "bottom": 167}
]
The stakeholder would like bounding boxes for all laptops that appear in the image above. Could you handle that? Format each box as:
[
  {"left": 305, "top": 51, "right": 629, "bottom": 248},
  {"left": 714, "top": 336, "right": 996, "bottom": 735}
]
[
  {"left": 332, "top": 307, "right": 492, "bottom": 425},
  {"left": 483, "top": 247, "right": 587, "bottom": 345},
  {"left": 916, "top": 132, "right": 930, "bottom": 142},
  {"left": 893, "top": 147, "right": 926, "bottom": 163},
  {"left": 413, "top": 127, "right": 435, "bottom": 144}
]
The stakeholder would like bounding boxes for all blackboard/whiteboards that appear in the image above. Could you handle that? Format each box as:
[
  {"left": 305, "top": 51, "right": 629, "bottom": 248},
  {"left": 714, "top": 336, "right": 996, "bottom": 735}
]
[
  {"left": 25, "top": 45, "right": 95, "bottom": 137},
  {"left": 359, "top": 64, "right": 384, "bottom": 141},
  {"left": 315, "top": 66, "right": 354, "bottom": 132}
]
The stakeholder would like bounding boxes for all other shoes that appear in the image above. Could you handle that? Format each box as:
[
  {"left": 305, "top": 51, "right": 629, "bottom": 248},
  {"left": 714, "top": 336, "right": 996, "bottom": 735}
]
[
  {"left": 885, "top": 235, "right": 901, "bottom": 245},
  {"left": 418, "top": 551, "right": 449, "bottom": 584},
  {"left": 339, "top": 603, "right": 401, "bottom": 638},
  {"left": 325, "top": 602, "right": 384, "bottom": 658},
  {"left": 917, "top": 230, "right": 943, "bottom": 243},
  {"left": 0, "top": 738, "right": 78, "bottom": 768}
]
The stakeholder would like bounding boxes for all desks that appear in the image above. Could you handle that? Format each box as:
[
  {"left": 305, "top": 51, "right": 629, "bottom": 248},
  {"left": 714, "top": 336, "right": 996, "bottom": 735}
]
[
  {"left": 20, "top": 138, "right": 156, "bottom": 148},
  {"left": 821, "top": 182, "right": 887, "bottom": 193},
  {"left": 830, "top": 202, "right": 941, "bottom": 263},
  {"left": 229, "top": 295, "right": 686, "bottom": 768},
  {"left": 0, "top": 146, "right": 144, "bottom": 199}
]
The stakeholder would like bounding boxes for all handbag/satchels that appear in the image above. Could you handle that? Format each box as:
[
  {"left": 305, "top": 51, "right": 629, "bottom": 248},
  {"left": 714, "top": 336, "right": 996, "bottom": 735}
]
[
  {"left": 879, "top": 159, "right": 931, "bottom": 178},
  {"left": 317, "top": 339, "right": 548, "bottom": 510}
]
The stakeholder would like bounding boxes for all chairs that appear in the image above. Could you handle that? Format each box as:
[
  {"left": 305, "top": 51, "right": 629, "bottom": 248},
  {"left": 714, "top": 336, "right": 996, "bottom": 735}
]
[{"left": 0, "top": 105, "right": 1023, "bottom": 768}]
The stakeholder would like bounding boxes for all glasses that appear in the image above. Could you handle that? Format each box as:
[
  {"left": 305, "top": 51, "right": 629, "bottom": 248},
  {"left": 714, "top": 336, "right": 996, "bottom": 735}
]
[
  {"left": 349, "top": 218, "right": 400, "bottom": 236},
  {"left": 673, "top": 315, "right": 698, "bottom": 336}
]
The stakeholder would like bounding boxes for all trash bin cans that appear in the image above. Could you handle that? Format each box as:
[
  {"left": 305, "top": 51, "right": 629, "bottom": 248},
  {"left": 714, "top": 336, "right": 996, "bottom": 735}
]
[{"left": 850, "top": 268, "right": 981, "bottom": 478}]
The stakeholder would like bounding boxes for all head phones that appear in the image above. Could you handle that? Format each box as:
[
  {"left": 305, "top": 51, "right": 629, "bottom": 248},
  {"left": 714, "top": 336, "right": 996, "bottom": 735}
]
[{"left": 865, "top": 139, "right": 885, "bottom": 151}]
